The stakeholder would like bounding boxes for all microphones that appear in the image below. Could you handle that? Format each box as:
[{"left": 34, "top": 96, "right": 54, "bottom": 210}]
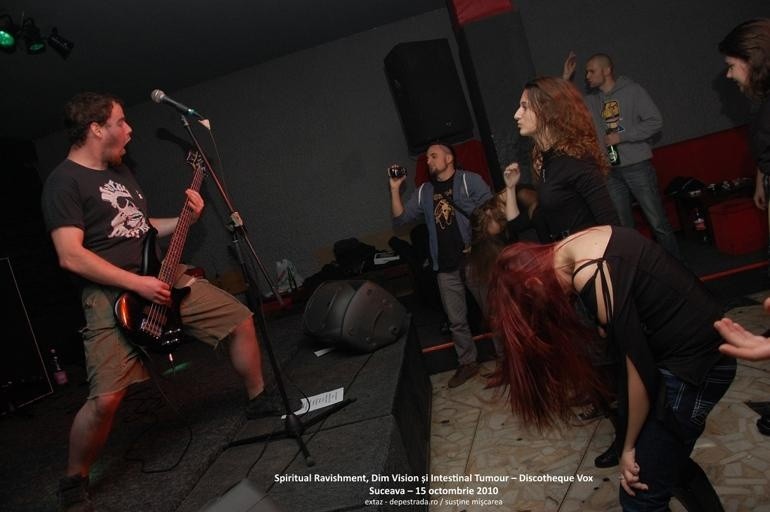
[{"left": 150, "top": 88, "right": 205, "bottom": 121}]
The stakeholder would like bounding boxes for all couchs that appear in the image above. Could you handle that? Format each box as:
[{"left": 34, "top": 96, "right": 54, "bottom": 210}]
[{"left": 634, "top": 125, "right": 770, "bottom": 302}]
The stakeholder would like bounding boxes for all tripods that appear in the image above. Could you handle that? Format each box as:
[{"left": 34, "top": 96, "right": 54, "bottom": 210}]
[{"left": 177, "top": 121, "right": 357, "bottom": 468}]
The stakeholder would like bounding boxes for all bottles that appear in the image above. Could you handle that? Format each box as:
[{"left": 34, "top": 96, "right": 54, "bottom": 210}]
[
  {"left": 46, "top": 347, "right": 68, "bottom": 393},
  {"left": 606, "top": 130, "right": 620, "bottom": 165},
  {"left": 690, "top": 206, "right": 708, "bottom": 242}
]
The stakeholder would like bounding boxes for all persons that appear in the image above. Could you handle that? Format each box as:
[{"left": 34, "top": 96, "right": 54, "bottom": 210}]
[
  {"left": 386, "top": 50, "right": 738, "bottom": 512},
  {"left": 718, "top": 17, "right": 770, "bottom": 235},
  {"left": 40, "top": 91, "right": 303, "bottom": 512},
  {"left": 711, "top": 297, "right": 770, "bottom": 364}
]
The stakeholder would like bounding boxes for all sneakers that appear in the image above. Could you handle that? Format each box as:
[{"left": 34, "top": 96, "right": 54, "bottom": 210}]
[
  {"left": 244, "top": 391, "right": 303, "bottom": 420},
  {"left": 56, "top": 473, "right": 93, "bottom": 512},
  {"left": 448, "top": 362, "right": 482, "bottom": 388},
  {"left": 569, "top": 403, "right": 608, "bottom": 426}
]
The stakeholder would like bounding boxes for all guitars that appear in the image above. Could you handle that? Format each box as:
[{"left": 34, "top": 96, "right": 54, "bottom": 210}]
[{"left": 113, "top": 151, "right": 211, "bottom": 349}]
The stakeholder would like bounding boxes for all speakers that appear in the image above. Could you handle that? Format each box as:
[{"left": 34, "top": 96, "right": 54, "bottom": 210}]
[
  {"left": 383, "top": 38, "right": 479, "bottom": 154},
  {"left": 303, "top": 280, "right": 410, "bottom": 353},
  {"left": 0, "top": 252, "right": 55, "bottom": 415}
]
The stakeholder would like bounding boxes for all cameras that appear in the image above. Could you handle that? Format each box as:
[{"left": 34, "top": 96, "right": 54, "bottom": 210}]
[{"left": 386, "top": 165, "right": 407, "bottom": 178}]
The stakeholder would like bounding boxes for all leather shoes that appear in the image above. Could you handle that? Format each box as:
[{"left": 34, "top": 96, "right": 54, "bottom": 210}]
[{"left": 595, "top": 445, "right": 618, "bottom": 468}]
